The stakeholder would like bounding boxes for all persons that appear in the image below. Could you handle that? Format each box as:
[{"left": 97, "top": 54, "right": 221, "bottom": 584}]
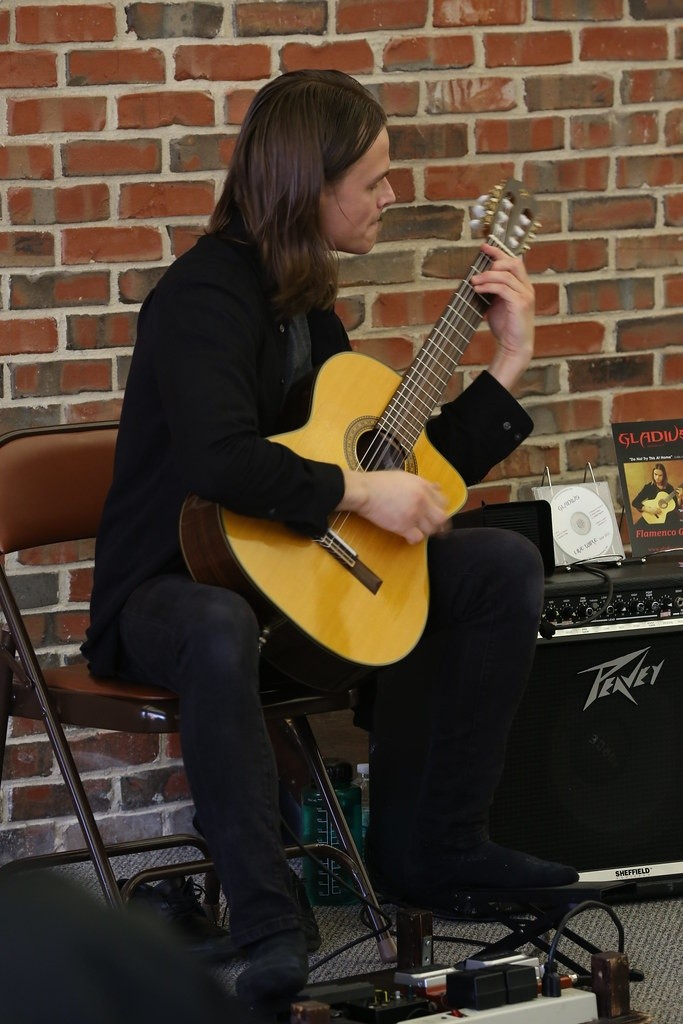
[
  {"left": 631, "top": 463, "right": 683, "bottom": 531},
  {"left": 80, "top": 67, "right": 578, "bottom": 1003}
]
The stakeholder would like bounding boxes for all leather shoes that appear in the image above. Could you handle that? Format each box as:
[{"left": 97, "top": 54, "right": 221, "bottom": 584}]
[{"left": 118, "top": 874, "right": 237, "bottom": 958}]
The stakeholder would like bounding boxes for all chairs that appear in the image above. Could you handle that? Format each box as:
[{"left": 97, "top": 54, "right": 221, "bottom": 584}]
[{"left": 1, "top": 419, "right": 401, "bottom": 968}]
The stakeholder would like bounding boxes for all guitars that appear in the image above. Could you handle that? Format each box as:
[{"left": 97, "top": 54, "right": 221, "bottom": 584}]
[{"left": 180, "top": 175, "right": 546, "bottom": 695}]
